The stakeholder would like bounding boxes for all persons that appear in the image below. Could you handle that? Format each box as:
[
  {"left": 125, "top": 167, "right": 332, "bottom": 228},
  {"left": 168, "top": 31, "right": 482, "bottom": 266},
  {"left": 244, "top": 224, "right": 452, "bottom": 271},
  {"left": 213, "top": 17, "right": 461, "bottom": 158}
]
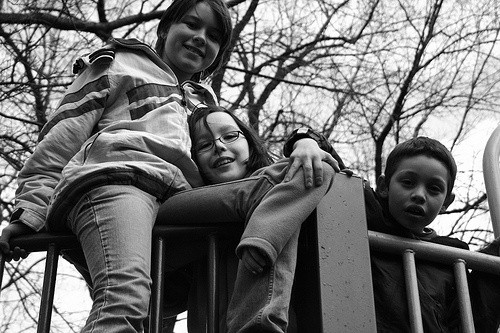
[
  {"left": 0, "top": 0, "right": 234, "bottom": 333},
  {"left": 187, "top": 106, "right": 337, "bottom": 333},
  {"left": 283, "top": 128, "right": 500, "bottom": 333}
]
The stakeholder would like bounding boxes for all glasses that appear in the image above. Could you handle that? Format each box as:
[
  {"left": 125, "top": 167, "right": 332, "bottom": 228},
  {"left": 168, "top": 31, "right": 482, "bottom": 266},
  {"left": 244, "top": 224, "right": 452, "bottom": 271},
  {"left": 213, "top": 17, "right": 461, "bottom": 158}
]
[{"left": 191, "top": 130, "right": 246, "bottom": 152}]
